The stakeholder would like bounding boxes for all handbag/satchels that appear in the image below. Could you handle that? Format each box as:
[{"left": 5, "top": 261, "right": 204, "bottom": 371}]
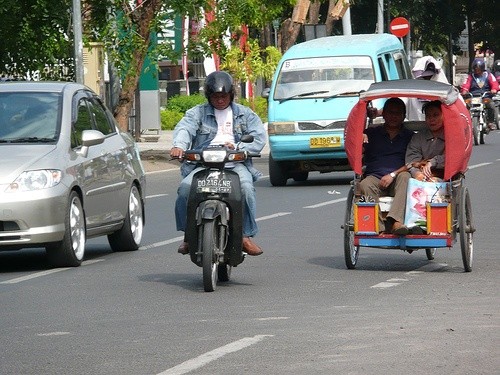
[{"left": 404, "top": 175, "right": 447, "bottom": 231}]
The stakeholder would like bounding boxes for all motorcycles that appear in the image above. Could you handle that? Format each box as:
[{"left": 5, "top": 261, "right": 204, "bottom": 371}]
[
  {"left": 461, "top": 90, "right": 496, "bottom": 146},
  {"left": 168, "top": 134, "right": 261, "bottom": 293}
]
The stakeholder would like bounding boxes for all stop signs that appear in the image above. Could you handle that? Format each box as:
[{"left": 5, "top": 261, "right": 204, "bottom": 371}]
[{"left": 389, "top": 17, "right": 410, "bottom": 37}]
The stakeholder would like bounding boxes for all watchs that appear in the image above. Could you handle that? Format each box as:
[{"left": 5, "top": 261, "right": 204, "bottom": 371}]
[{"left": 389, "top": 172, "right": 397, "bottom": 180}]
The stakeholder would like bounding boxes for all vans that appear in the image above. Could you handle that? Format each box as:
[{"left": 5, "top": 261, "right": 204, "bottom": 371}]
[{"left": 261, "top": 33, "right": 412, "bottom": 185}]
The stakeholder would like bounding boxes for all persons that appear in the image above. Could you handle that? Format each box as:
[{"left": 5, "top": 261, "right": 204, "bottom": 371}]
[
  {"left": 359, "top": 97, "right": 415, "bottom": 235},
  {"left": 460, "top": 57, "right": 500, "bottom": 130},
  {"left": 169, "top": 70, "right": 267, "bottom": 257},
  {"left": 404, "top": 99, "right": 444, "bottom": 235}
]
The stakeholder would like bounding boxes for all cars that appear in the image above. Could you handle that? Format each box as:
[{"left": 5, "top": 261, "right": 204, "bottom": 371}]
[{"left": 0, "top": 81, "right": 146, "bottom": 266}]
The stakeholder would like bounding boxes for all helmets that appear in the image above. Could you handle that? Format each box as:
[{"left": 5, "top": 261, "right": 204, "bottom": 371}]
[
  {"left": 472, "top": 58, "right": 485, "bottom": 71},
  {"left": 491, "top": 60, "right": 500, "bottom": 72},
  {"left": 204, "top": 70, "right": 236, "bottom": 110}
]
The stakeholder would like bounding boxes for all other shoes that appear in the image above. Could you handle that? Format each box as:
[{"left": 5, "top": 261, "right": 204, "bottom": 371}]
[{"left": 411, "top": 225, "right": 423, "bottom": 235}]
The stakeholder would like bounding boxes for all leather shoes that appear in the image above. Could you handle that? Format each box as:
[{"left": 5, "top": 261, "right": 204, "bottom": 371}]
[
  {"left": 177, "top": 241, "right": 188, "bottom": 254},
  {"left": 242, "top": 237, "right": 264, "bottom": 256},
  {"left": 390, "top": 221, "right": 409, "bottom": 235}
]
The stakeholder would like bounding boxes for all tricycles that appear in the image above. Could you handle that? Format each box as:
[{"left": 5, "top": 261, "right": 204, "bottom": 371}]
[{"left": 341, "top": 78, "right": 476, "bottom": 272}]
